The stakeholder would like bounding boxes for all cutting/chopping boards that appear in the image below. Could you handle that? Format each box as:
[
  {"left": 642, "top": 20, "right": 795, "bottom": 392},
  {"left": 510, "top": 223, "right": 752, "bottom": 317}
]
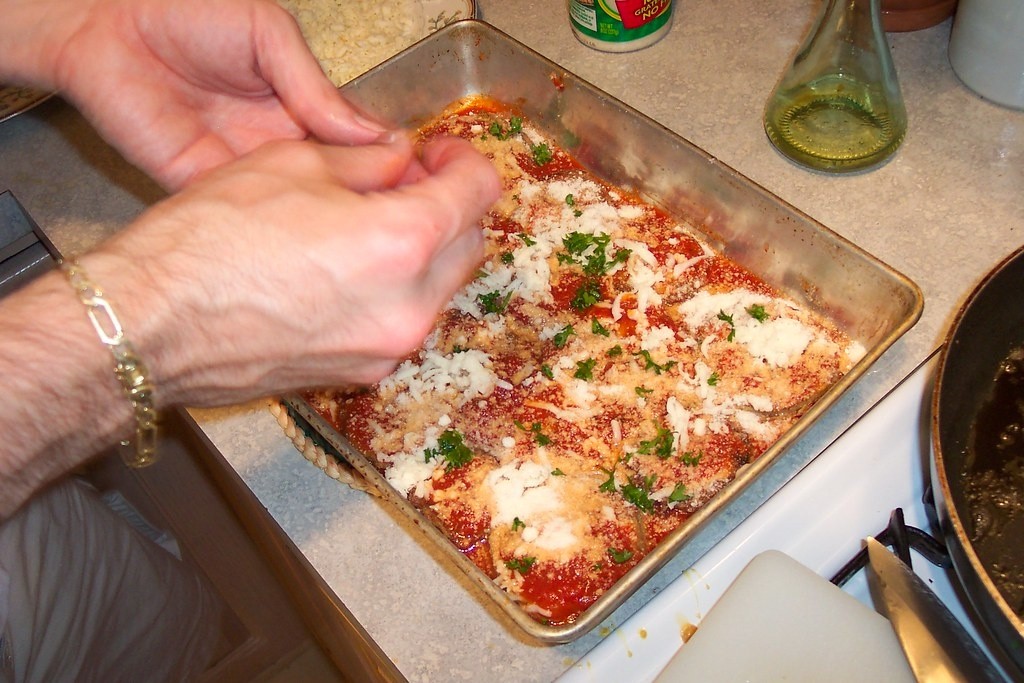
[{"left": 652, "top": 549, "right": 916, "bottom": 683}]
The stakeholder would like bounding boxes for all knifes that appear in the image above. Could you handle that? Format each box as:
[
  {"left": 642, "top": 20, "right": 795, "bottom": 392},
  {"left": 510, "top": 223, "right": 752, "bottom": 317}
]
[{"left": 863, "top": 535, "right": 1006, "bottom": 682}]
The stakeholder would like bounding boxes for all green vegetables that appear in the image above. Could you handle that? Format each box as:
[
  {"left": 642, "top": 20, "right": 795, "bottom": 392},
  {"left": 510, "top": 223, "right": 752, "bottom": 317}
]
[{"left": 424, "top": 116, "right": 768, "bottom": 573}]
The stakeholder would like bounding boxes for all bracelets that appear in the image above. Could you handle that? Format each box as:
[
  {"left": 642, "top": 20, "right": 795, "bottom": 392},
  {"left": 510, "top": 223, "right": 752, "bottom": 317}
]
[{"left": 55, "top": 258, "right": 160, "bottom": 470}]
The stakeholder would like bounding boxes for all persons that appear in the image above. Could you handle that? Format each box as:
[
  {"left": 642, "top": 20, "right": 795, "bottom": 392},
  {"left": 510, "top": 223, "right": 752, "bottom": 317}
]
[{"left": 0, "top": 1, "right": 502, "bottom": 683}]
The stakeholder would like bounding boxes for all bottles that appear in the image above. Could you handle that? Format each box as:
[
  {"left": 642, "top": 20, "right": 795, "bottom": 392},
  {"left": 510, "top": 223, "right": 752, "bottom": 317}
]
[
  {"left": 762, "top": 0, "right": 908, "bottom": 174},
  {"left": 567, "top": 0, "right": 675, "bottom": 54},
  {"left": 879, "top": 1, "right": 955, "bottom": 33},
  {"left": 948, "top": 0, "right": 1024, "bottom": 110}
]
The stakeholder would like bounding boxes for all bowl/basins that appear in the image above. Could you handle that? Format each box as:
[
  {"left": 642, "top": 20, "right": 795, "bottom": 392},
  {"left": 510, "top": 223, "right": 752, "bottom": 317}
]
[{"left": 928, "top": 246, "right": 1024, "bottom": 682}]
